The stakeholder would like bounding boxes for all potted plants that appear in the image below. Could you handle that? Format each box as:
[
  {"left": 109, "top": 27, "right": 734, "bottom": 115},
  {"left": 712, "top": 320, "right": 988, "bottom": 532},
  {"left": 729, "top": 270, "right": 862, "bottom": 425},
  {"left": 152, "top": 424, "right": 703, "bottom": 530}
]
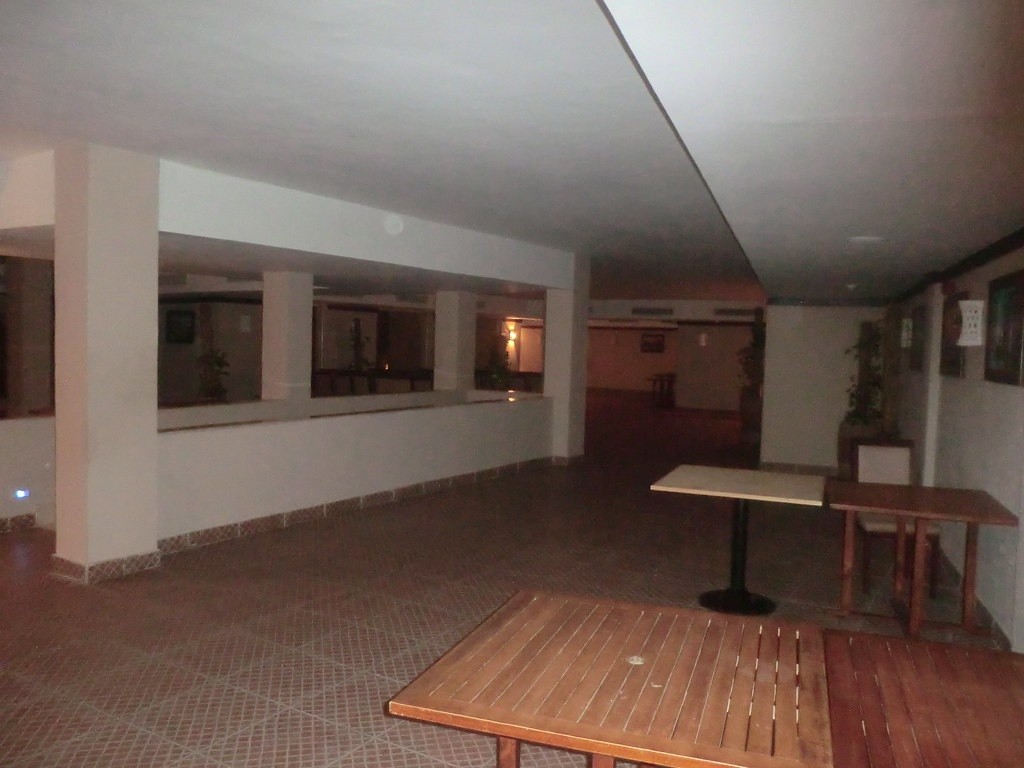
[{"left": 839, "top": 319, "right": 884, "bottom": 475}]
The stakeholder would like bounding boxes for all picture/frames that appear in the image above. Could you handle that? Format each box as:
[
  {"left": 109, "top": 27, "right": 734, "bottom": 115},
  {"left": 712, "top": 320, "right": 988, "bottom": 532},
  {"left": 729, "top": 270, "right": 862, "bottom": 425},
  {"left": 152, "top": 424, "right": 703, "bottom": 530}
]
[
  {"left": 940, "top": 291, "right": 970, "bottom": 378},
  {"left": 984, "top": 268, "right": 1024, "bottom": 386}
]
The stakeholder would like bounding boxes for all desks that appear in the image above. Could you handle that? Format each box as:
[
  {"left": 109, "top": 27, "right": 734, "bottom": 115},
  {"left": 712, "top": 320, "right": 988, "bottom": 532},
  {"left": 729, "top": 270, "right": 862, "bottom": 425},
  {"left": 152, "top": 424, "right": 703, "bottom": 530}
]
[
  {"left": 851, "top": 435, "right": 914, "bottom": 483},
  {"left": 651, "top": 464, "right": 826, "bottom": 615},
  {"left": 823, "top": 628, "right": 1024, "bottom": 768},
  {"left": 823, "top": 479, "right": 1019, "bottom": 642},
  {"left": 389, "top": 591, "right": 833, "bottom": 768},
  {"left": 320, "top": 368, "right": 433, "bottom": 393}
]
